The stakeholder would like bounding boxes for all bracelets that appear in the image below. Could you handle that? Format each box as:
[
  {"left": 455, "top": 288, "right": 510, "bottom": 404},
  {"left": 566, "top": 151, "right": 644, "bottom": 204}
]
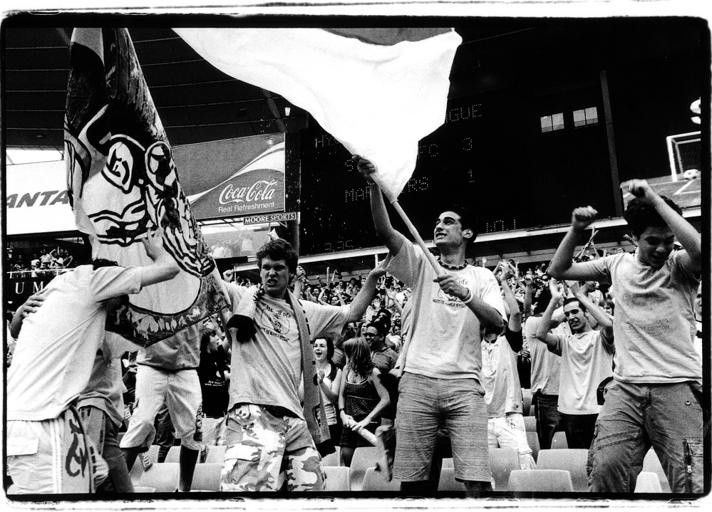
[{"left": 462, "top": 288, "right": 471, "bottom": 301}]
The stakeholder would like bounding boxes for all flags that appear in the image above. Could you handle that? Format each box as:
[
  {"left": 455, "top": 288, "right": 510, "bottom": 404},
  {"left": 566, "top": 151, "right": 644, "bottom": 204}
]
[
  {"left": 172, "top": 27, "right": 467, "bottom": 201},
  {"left": 61, "top": 22, "right": 226, "bottom": 339}
]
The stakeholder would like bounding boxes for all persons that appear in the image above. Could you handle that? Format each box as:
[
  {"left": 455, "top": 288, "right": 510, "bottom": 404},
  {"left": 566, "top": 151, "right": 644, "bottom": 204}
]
[
  {"left": 8, "top": 226, "right": 180, "bottom": 497},
  {"left": 478, "top": 245, "right": 626, "bottom": 470},
  {"left": 357, "top": 159, "right": 509, "bottom": 491},
  {"left": 117, "top": 272, "right": 254, "bottom": 492},
  {"left": 199, "top": 236, "right": 392, "bottom": 497},
  {"left": 290, "top": 265, "right": 406, "bottom": 467},
  {"left": 6, "top": 242, "right": 73, "bottom": 273},
  {"left": 544, "top": 176, "right": 704, "bottom": 494},
  {"left": 693, "top": 286, "right": 702, "bottom": 356},
  {"left": 10, "top": 287, "right": 139, "bottom": 496}
]
